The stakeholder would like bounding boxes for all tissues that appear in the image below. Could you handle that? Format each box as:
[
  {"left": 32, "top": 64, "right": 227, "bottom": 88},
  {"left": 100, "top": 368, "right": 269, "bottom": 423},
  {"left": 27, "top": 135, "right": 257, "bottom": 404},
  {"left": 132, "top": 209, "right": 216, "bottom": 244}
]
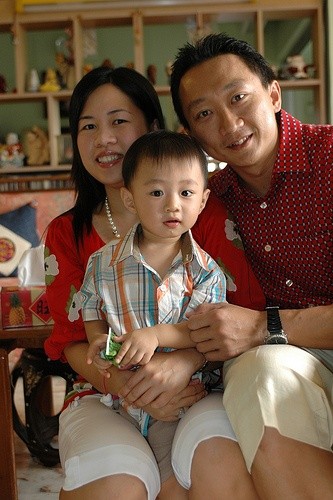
[{"left": 0, "top": 244, "right": 55, "bottom": 329}]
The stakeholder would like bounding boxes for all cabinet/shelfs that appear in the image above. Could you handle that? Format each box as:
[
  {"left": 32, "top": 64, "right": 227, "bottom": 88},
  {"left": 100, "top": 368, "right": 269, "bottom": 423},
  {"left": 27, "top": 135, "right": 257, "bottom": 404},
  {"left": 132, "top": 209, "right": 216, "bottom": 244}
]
[{"left": 0, "top": 0, "right": 333, "bottom": 172}]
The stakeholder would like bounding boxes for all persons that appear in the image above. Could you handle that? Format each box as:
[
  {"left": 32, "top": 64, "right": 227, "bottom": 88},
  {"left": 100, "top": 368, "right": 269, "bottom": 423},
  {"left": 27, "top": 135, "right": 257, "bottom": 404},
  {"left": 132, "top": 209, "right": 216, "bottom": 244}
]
[
  {"left": 42, "top": 65, "right": 258, "bottom": 500},
  {"left": 169, "top": 31, "right": 332, "bottom": 500},
  {"left": 78, "top": 129, "right": 228, "bottom": 500}
]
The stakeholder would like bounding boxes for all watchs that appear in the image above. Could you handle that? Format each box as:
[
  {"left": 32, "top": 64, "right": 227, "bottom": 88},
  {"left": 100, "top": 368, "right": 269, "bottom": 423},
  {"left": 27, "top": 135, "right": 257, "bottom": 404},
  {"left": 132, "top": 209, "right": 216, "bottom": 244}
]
[{"left": 263, "top": 305, "right": 288, "bottom": 345}]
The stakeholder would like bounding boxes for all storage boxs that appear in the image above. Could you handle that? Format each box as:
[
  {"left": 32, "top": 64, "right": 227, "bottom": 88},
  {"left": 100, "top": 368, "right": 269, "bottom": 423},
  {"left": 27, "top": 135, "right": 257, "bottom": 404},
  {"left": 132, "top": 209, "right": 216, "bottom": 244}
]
[{"left": 0, "top": 276, "right": 57, "bottom": 328}]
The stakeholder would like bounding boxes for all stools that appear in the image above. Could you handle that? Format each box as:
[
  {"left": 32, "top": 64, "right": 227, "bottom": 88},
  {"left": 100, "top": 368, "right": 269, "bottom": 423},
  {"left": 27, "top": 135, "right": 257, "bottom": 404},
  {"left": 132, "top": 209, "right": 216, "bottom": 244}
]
[{"left": 7, "top": 348, "right": 83, "bottom": 468}]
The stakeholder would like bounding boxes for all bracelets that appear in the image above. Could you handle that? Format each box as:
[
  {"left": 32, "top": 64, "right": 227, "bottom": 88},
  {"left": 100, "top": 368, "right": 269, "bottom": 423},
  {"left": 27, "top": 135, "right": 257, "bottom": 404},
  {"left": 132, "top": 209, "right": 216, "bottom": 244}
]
[{"left": 100, "top": 363, "right": 115, "bottom": 408}]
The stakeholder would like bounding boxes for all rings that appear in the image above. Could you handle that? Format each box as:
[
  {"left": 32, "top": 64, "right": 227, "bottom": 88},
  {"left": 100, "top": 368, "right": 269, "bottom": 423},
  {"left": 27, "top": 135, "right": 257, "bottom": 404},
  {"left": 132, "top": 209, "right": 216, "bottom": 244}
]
[{"left": 178, "top": 407, "right": 184, "bottom": 419}]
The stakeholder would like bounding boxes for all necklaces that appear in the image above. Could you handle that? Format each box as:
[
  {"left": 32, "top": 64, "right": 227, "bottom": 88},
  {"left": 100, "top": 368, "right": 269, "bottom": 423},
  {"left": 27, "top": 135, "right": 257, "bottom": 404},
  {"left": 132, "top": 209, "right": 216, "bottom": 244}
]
[{"left": 105, "top": 196, "right": 124, "bottom": 241}]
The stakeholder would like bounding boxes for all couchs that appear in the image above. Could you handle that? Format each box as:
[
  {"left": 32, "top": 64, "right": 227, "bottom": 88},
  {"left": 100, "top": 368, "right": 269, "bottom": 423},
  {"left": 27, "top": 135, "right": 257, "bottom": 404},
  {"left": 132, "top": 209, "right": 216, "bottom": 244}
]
[{"left": 0, "top": 175, "right": 79, "bottom": 241}]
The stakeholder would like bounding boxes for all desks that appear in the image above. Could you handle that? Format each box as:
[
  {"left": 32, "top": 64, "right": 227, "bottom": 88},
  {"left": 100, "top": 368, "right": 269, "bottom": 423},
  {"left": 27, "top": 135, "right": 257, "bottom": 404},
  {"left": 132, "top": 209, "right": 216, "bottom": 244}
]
[{"left": 0, "top": 323, "right": 59, "bottom": 500}]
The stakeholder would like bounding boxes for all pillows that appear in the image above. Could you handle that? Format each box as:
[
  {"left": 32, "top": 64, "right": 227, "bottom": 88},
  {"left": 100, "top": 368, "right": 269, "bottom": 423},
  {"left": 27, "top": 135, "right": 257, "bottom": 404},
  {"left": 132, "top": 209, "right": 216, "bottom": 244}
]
[{"left": 1, "top": 200, "right": 40, "bottom": 278}]
